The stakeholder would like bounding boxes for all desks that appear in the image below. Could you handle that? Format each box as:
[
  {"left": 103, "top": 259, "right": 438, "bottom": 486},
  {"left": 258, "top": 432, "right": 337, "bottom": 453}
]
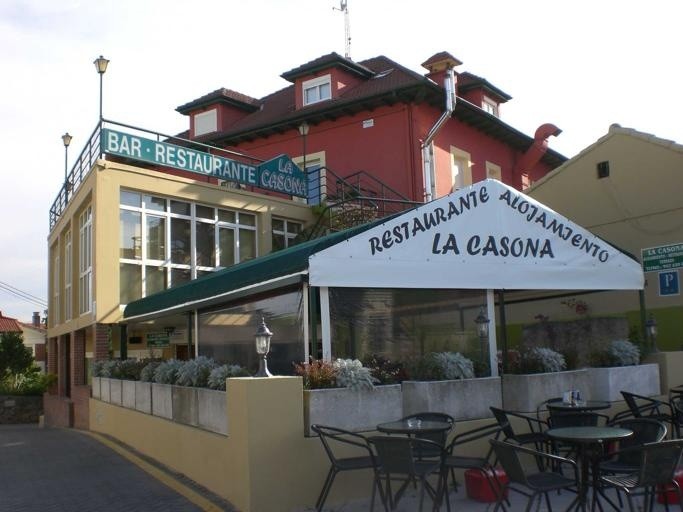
[
  {"left": 546, "top": 402, "right": 610, "bottom": 425},
  {"left": 378, "top": 422, "right": 451, "bottom": 434},
  {"left": 548, "top": 426, "right": 621, "bottom": 512},
  {"left": 547, "top": 402, "right": 610, "bottom": 411}
]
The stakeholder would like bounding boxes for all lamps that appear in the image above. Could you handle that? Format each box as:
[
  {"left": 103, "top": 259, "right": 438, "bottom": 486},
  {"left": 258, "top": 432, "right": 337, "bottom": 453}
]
[
  {"left": 645, "top": 314, "right": 661, "bottom": 353},
  {"left": 475, "top": 305, "right": 490, "bottom": 338},
  {"left": 252, "top": 316, "right": 273, "bottom": 377}
]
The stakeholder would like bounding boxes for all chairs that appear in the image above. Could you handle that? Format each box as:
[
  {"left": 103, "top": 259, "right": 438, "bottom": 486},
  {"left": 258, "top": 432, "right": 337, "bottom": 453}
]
[
  {"left": 386, "top": 413, "right": 460, "bottom": 495},
  {"left": 490, "top": 406, "right": 565, "bottom": 478},
  {"left": 596, "top": 416, "right": 667, "bottom": 509},
  {"left": 366, "top": 435, "right": 450, "bottom": 511},
  {"left": 311, "top": 424, "right": 382, "bottom": 511},
  {"left": 670, "top": 385, "right": 682, "bottom": 438},
  {"left": 537, "top": 398, "right": 564, "bottom": 431},
  {"left": 620, "top": 391, "right": 682, "bottom": 439},
  {"left": 487, "top": 438, "right": 584, "bottom": 512},
  {"left": 592, "top": 439, "right": 681, "bottom": 511},
  {"left": 435, "top": 422, "right": 512, "bottom": 511}
]
